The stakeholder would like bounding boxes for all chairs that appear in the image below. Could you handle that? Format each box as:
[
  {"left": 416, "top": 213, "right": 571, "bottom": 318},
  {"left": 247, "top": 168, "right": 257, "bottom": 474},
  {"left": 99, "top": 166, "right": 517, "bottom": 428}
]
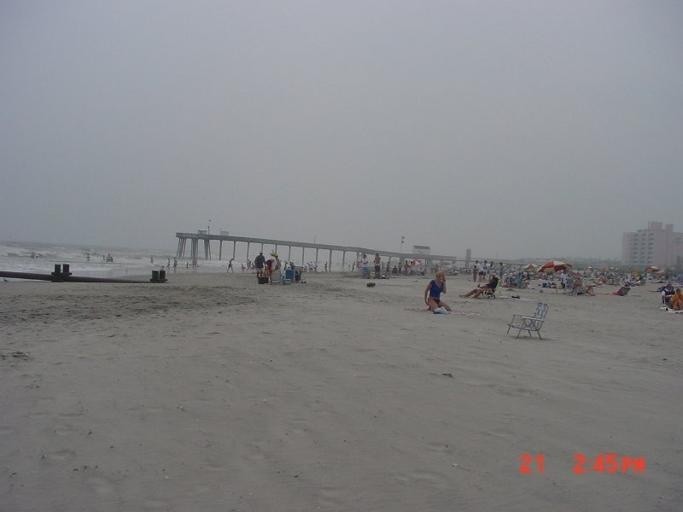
[
  {"left": 658, "top": 285, "right": 683, "bottom": 313},
  {"left": 506, "top": 303, "right": 549, "bottom": 340},
  {"left": 477, "top": 283, "right": 499, "bottom": 299}
]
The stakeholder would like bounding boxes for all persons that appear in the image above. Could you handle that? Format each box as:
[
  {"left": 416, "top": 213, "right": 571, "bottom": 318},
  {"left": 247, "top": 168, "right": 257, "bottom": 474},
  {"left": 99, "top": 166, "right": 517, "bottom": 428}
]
[
  {"left": 661, "top": 284, "right": 676, "bottom": 306},
  {"left": 423, "top": 272, "right": 452, "bottom": 312},
  {"left": 149, "top": 255, "right": 199, "bottom": 273},
  {"left": 253, "top": 253, "right": 265, "bottom": 278},
  {"left": 459, "top": 274, "right": 498, "bottom": 299},
  {"left": 263, "top": 259, "right": 274, "bottom": 285},
  {"left": 471, "top": 259, "right": 683, "bottom": 298},
  {"left": 671, "top": 288, "right": 683, "bottom": 310},
  {"left": 303, "top": 260, "right": 327, "bottom": 273},
  {"left": 373, "top": 253, "right": 381, "bottom": 278},
  {"left": 226, "top": 257, "right": 254, "bottom": 274},
  {"left": 360, "top": 254, "right": 370, "bottom": 279},
  {"left": 386, "top": 259, "right": 425, "bottom": 276}
]
